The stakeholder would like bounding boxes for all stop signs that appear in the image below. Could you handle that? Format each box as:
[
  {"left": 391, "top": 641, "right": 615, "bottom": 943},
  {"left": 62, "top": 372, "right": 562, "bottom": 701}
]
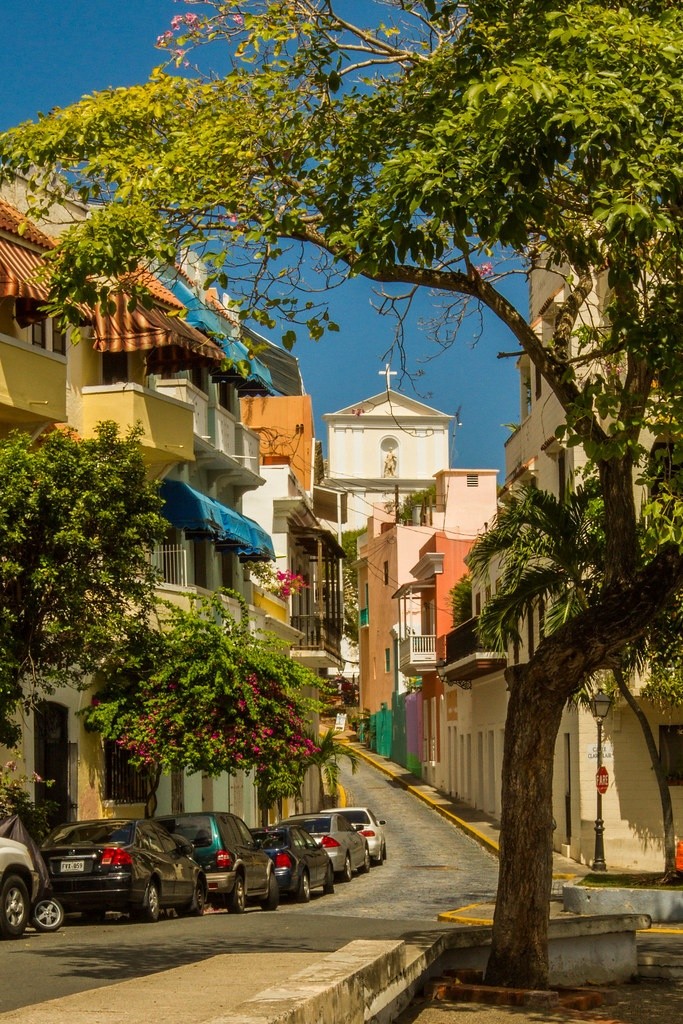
[{"left": 595, "top": 766, "right": 610, "bottom": 795}]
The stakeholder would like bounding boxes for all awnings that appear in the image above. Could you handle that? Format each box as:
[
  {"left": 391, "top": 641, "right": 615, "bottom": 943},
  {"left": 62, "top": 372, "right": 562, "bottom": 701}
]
[
  {"left": 84, "top": 271, "right": 304, "bottom": 401},
  {"left": 159, "top": 479, "right": 276, "bottom": 561},
  {"left": 0, "top": 241, "right": 90, "bottom": 325}
]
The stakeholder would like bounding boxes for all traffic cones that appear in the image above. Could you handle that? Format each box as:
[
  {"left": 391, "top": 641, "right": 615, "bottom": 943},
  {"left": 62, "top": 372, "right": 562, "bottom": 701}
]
[{"left": 674, "top": 838, "right": 683, "bottom": 872}]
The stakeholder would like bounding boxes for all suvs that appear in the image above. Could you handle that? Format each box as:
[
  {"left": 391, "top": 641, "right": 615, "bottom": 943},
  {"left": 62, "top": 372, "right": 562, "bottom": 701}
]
[{"left": 146, "top": 811, "right": 280, "bottom": 914}]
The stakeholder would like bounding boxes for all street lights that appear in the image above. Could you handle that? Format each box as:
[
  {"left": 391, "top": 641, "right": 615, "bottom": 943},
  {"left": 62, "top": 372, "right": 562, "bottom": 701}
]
[{"left": 589, "top": 687, "right": 613, "bottom": 871}]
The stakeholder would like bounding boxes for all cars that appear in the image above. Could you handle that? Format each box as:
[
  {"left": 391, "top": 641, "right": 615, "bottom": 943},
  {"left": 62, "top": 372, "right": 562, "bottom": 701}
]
[
  {"left": 246, "top": 824, "right": 335, "bottom": 904},
  {"left": 0, "top": 835, "right": 40, "bottom": 939},
  {"left": 37, "top": 817, "right": 209, "bottom": 924},
  {"left": 321, "top": 806, "right": 387, "bottom": 867},
  {"left": 274, "top": 812, "right": 371, "bottom": 883}
]
